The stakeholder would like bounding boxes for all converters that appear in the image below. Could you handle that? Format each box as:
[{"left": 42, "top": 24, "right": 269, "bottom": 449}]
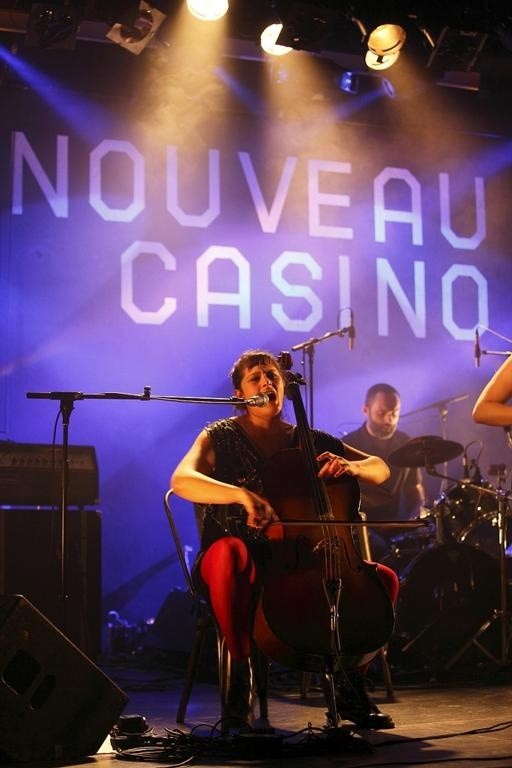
[
  {"left": 238, "top": 733, "right": 283, "bottom": 746},
  {"left": 116, "top": 714, "right": 144, "bottom": 732}
]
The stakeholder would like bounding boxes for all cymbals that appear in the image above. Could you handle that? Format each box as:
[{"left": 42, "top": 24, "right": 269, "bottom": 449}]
[{"left": 388, "top": 440, "right": 464, "bottom": 467}]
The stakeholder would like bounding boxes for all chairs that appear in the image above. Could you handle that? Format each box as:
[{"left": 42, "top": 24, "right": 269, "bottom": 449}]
[{"left": 158, "top": 485, "right": 342, "bottom": 737}]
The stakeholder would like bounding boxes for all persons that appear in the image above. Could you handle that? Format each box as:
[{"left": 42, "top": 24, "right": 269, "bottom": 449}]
[
  {"left": 339, "top": 381, "right": 425, "bottom": 540},
  {"left": 471, "top": 352, "right": 512, "bottom": 427},
  {"left": 169, "top": 349, "right": 398, "bottom": 737}
]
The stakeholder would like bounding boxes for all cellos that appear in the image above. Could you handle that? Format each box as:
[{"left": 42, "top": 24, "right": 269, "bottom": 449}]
[{"left": 251, "top": 351, "right": 396, "bottom": 674}]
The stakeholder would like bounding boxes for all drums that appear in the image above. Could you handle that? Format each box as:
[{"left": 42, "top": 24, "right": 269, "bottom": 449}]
[
  {"left": 381, "top": 531, "right": 500, "bottom": 670},
  {"left": 432, "top": 478, "right": 511, "bottom": 542}
]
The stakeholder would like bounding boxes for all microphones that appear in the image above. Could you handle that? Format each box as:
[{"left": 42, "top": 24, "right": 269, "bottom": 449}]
[
  {"left": 463, "top": 455, "right": 469, "bottom": 479},
  {"left": 247, "top": 392, "right": 269, "bottom": 407},
  {"left": 349, "top": 315, "right": 355, "bottom": 351},
  {"left": 475, "top": 330, "right": 480, "bottom": 367}
]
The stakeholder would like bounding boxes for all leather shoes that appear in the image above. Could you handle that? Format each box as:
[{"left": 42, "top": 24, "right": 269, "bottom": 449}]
[
  {"left": 227, "top": 665, "right": 253, "bottom": 711},
  {"left": 329, "top": 693, "right": 395, "bottom": 728}
]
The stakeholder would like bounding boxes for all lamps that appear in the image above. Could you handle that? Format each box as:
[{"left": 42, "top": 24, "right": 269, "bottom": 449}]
[{"left": 25, "top": 1, "right": 491, "bottom": 93}]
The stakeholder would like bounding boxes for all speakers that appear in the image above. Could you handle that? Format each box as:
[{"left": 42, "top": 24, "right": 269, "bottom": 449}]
[
  {"left": 0, "top": 511, "right": 102, "bottom": 658},
  {"left": 140, "top": 586, "right": 197, "bottom": 652},
  {"left": 0, "top": 594, "right": 128, "bottom": 768}
]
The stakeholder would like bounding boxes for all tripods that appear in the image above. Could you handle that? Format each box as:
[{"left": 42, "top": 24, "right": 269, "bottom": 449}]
[{"left": 400, "top": 497, "right": 512, "bottom": 683}]
